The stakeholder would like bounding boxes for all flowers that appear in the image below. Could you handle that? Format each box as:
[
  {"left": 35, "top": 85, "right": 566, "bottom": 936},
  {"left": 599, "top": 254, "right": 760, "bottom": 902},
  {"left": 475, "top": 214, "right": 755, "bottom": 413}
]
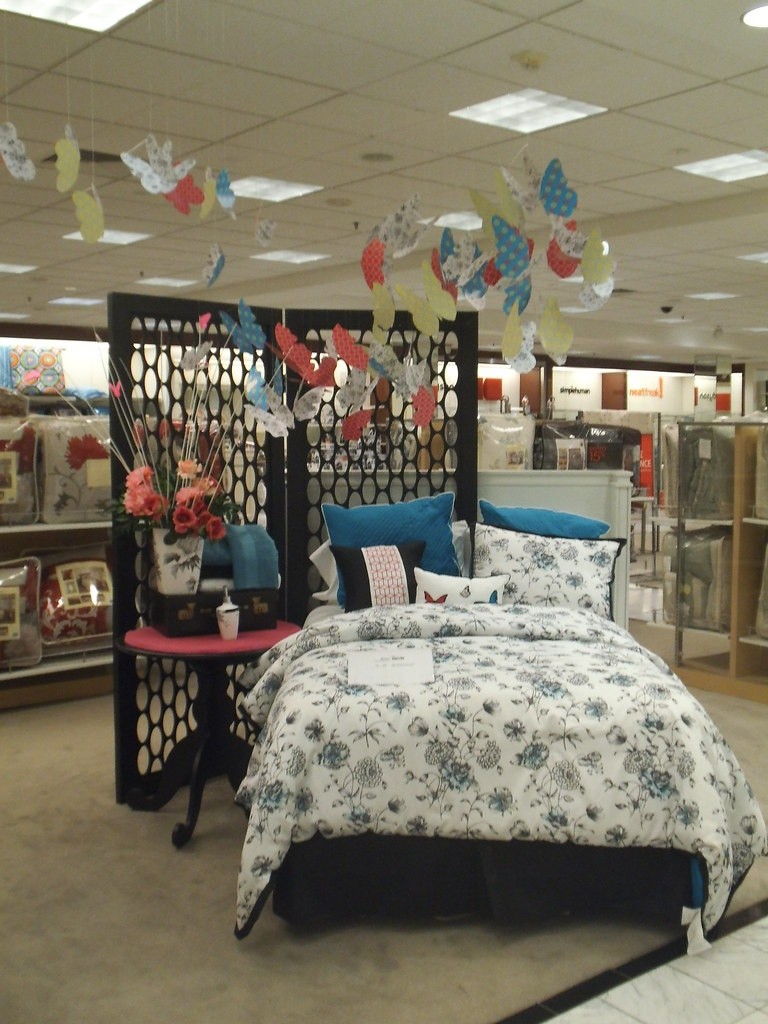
[{"left": 123, "top": 459, "right": 244, "bottom": 546}]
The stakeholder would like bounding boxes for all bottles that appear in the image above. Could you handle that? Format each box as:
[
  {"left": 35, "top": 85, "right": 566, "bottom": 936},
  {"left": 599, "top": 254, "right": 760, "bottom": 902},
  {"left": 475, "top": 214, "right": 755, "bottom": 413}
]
[{"left": 215, "top": 585, "right": 241, "bottom": 642}]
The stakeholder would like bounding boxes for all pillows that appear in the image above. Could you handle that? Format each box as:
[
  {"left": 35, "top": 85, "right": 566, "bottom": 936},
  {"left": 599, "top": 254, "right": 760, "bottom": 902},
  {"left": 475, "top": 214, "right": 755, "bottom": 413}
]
[
  {"left": 468, "top": 519, "right": 627, "bottom": 623},
  {"left": 479, "top": 498, "right": 611, "bottom": 540},
  {"left": 320, "top": 491, "right": 463, "bottom": 608},
  {"left": 328, "top": 541, "right": 427, "bottom": 613},
  {"left": 413, "top": 567, "right": 512, "bottom": 605}
]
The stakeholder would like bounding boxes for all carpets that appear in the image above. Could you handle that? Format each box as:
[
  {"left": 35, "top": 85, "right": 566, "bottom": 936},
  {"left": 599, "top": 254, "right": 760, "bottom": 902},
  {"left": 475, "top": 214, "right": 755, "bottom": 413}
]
[{"left": 3, "top": 683, "right": 768, "bottom": 1022}]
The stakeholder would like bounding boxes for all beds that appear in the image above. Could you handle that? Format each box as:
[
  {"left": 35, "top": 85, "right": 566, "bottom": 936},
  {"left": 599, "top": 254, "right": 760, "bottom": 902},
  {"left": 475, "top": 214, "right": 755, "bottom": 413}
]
[{"left": 230, "top": 605, "right": 767, "bottom": 941}]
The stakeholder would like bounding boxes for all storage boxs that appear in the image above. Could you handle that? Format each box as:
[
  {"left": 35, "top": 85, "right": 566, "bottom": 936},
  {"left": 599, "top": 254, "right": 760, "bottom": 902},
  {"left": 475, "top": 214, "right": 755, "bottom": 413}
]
[{"left": 146, "top": 588, "right": 278, "bottom": 639}]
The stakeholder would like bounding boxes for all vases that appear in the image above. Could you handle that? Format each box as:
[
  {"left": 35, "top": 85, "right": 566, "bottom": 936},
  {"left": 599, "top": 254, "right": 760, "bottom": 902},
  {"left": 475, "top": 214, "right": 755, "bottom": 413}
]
[{"left": 153, "top": 527, "right": 206, "bottom": 596}]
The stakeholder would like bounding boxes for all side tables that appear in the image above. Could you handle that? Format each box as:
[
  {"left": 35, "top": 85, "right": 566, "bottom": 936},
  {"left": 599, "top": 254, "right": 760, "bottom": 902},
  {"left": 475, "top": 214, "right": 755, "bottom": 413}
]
[{"left": 114, "top": 620, "right": 306, "bottom": 851}]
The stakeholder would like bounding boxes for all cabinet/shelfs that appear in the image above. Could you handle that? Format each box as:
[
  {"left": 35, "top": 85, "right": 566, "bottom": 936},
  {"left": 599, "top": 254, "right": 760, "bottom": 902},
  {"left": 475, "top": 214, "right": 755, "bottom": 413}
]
[
  {"left": 1, "top": 320, "right": 748, "bottom": 712},
  {"left": 659, "top": 419, "right": 767, "bottom": 706}
]
